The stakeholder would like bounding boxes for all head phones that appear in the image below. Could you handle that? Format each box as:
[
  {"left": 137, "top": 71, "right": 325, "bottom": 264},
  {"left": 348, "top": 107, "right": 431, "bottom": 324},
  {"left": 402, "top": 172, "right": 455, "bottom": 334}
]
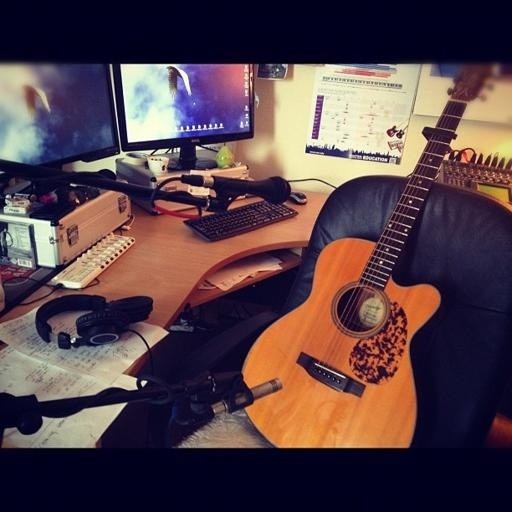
[{"left": 36, "top": 293, "right": 154, "bottom": 352}]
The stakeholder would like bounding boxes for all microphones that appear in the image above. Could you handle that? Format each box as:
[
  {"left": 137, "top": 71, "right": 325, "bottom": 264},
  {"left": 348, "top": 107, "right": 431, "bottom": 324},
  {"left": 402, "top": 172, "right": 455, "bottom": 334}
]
[
  {"left": 180, "top": 173, "right": 292, "bottom": 206},
  {"left": 209, "top": 377, "right": 283, "bottom": 416}
]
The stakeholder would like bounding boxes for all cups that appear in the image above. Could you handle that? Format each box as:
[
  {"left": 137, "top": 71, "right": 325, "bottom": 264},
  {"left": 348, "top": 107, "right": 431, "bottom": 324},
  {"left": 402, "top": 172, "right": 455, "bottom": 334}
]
[{"left": 147, "top": 156, "right": 169, "bottom": 175}]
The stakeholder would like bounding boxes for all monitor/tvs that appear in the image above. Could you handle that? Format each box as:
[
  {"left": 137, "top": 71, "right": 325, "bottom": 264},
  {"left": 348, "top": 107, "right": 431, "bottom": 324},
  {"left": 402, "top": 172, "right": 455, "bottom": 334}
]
[
  {"left": 0, "top": 61, "right": 121, "bottom": 173},
  {"left": 111, "top": 63, "right": 255, "bottom": 170}
]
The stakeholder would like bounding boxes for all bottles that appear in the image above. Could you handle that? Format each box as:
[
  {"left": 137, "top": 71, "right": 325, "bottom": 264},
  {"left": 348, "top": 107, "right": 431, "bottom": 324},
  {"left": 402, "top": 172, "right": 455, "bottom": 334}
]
[{"left": 215, "top": 146, "right": 233, "bottom": 168}]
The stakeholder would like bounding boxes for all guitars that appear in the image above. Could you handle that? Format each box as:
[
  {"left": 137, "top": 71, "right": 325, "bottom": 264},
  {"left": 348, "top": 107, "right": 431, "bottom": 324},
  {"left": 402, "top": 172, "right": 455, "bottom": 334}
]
[
  {"left": 385, "top": 119, "right": 407, "bottom": 139},
  {"left": 240, "top": 64, "right": 498, "bottom": 448}
]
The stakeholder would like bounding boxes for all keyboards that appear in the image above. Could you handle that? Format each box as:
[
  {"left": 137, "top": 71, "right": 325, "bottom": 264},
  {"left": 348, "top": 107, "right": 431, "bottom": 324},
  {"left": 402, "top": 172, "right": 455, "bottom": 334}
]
[
  {"left": 182, "top": 200, "right": 299, "bottom": 242},
  {"left": 52, "top": 232, "right": 135, "bottom": 290}
]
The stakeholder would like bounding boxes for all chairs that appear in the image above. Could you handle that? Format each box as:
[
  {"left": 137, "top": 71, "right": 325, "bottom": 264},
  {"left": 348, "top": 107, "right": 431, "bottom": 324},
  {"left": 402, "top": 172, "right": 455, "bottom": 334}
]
[{"left": 162, "top": 176, "right": 510, "bottom": 449}]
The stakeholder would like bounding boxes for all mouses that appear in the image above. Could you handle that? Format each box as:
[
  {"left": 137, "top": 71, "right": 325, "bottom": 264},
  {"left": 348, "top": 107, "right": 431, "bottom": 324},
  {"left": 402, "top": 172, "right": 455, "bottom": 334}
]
[{"left": 291, "top": 190, "right": 308, "bottom": 204}]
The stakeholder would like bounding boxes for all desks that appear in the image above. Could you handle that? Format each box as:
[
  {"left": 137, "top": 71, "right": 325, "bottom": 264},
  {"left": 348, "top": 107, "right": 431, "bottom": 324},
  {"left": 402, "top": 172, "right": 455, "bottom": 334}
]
[{"left": 0, "top": 184, "right": 332, "bottom": 447}]
